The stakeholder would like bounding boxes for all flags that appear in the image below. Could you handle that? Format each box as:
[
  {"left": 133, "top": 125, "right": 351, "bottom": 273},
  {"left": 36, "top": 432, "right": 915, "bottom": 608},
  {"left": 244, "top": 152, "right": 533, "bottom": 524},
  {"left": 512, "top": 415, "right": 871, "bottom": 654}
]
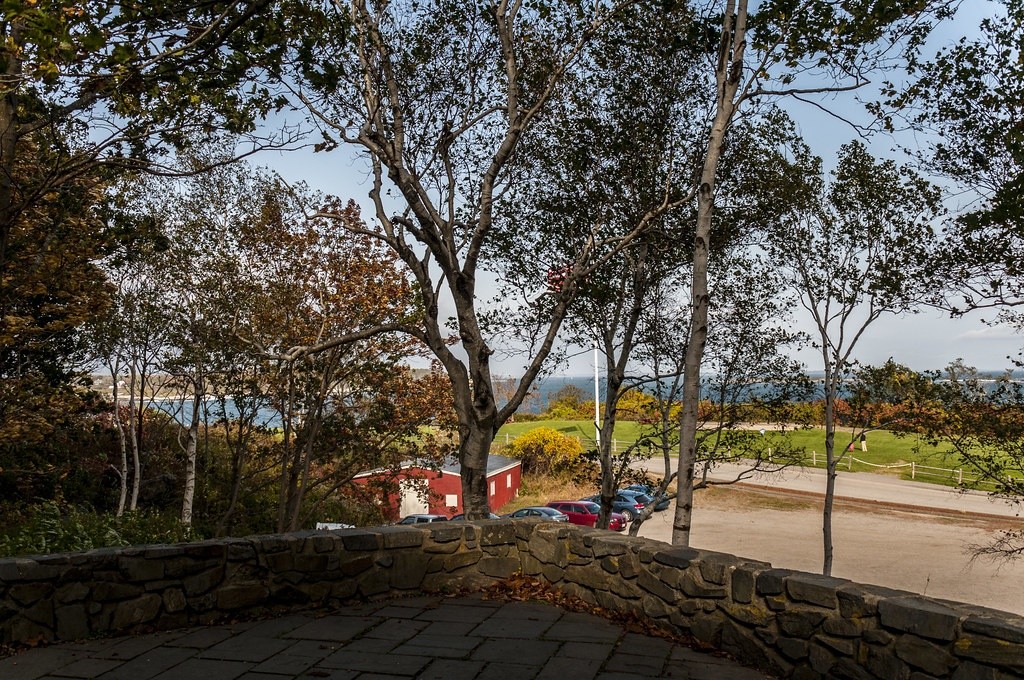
[{"left": 548, "top": 267, "right": 570, "bottom": 292}]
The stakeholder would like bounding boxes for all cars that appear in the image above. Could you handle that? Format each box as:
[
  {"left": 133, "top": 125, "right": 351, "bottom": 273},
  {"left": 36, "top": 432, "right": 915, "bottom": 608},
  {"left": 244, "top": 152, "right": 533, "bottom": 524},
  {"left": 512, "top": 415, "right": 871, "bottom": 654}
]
[
  {"left": 623, "top": 485, "right": 670, "bottom": 510},
  {"left": 500, "top": 506, "right": 570, "bottom": 522},
  {"left": 579, "top": 489, "right": 653, "bottom": 522},
  {"left": 450, "top": 512, "right": 499, "bottom": 522}
]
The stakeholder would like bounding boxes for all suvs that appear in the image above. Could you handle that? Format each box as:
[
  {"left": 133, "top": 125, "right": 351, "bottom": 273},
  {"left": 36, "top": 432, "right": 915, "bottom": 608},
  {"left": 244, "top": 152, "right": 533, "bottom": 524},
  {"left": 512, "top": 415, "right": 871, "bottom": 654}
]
[
  {"left": 382, "top": 514, "right": 448, "bottom": 526},
  {"left": 544, "top": 500, "right": 627, "bottom": 533}
]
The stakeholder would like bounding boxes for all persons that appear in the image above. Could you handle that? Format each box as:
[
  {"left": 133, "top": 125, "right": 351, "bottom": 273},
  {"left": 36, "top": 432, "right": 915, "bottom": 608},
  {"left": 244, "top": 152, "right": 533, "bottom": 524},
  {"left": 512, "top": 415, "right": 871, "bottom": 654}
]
[{"left": 860, "top": 433, "right": 868, "bottom": 451}]
